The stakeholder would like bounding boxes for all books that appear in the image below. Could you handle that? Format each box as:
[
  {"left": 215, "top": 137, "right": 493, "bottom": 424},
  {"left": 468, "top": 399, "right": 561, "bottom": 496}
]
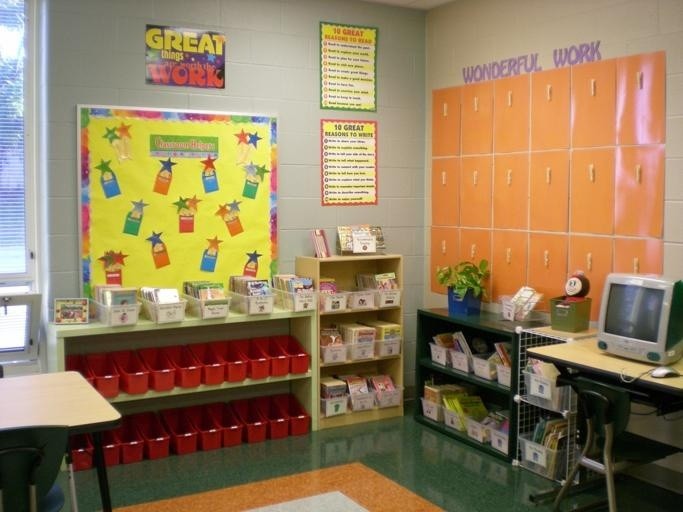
[
  {"left": 423, "top": 331, "right": 512, "bottom": 433},
  {"left": 94, "top": 274, "right": 313, "bottom": 306},
  {"left": 310, "top": 224, "right": 401, "bottom": 416},
  {"left": 526, "top": 416, "right": 585, "bottom": 478}
]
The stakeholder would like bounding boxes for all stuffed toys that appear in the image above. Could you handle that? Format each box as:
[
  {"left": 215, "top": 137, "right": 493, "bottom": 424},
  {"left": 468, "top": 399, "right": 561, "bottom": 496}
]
[{"left": 557, "top": 270, "right": 590, "bottom": 307}]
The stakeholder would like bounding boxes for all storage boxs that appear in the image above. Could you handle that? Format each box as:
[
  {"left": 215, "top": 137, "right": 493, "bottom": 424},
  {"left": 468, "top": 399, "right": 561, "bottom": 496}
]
[{"left": 64, "top": 334, "right": 310, "bottom": 473}]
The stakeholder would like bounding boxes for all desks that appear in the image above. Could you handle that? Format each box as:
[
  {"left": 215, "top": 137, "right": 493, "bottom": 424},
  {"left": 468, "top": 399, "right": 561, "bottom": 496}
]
[
  {"left": 0, "top": 370, "right": 122, "bottom": 512},
  {"left": 526, "top": 336, "right": 683, "bottom": 507}
]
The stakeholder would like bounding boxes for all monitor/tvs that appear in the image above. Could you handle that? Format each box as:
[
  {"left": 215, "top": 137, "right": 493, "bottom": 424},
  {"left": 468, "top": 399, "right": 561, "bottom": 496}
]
[{"left": 597, "top": 272, "right": 683, "bottom": 366}]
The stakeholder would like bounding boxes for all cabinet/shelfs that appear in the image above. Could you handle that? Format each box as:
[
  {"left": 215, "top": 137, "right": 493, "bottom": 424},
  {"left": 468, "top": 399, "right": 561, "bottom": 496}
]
[
  {"left": 47, "top": 305, "right": 317, "bottom": 472},
  {"left": 414, "top": 308, "right": 514, "bottom": 461},
  {"left": 296, "top": 253, "right": 404, "bottom": 428},
  {"left": 512, "top": 326, "right": 580, "bottom": 486}
]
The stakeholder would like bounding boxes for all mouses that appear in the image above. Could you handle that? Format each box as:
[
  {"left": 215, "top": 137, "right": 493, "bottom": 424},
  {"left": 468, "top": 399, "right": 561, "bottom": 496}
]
[{"left": 651, "top": 366, "right": 681, "bottom": 378}]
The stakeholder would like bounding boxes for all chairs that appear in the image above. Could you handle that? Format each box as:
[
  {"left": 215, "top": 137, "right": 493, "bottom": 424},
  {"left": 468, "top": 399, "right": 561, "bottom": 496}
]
[
  {"left": 551, "top": 373, "right": 683, "bottom": 512},
  {"left": 0, "top": 425, "right": 69, "bottom": 511}
]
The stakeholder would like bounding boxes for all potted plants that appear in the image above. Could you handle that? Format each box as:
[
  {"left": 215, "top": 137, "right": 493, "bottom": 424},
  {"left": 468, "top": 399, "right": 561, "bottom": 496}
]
[{"left": 437, "top": 259, "right": 489, "bottom": 315}]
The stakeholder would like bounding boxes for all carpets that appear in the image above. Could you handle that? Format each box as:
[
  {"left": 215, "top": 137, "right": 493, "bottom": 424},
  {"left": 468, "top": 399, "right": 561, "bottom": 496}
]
[{"left": 113, "top": 461, "right": 446, "bottom": 512}]
